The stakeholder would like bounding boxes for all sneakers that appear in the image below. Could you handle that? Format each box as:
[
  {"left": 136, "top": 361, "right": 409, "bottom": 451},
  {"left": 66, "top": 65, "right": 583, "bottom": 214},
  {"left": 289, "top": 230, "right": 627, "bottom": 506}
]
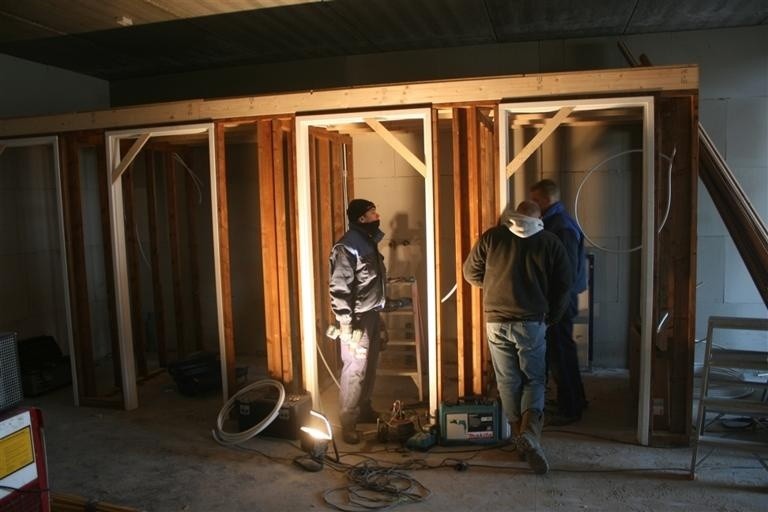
[
  {"left": 515, "top": 432, "right": 548, "bottom": 475},
  {"left": 541, "top": 399, "right": 586, "bottom": 426},
  {"left": 358, "top": 411, "right": 389, "bottom": 422},
  {"left": 341, "top": 423, "right": 361, "bottom": 445}
]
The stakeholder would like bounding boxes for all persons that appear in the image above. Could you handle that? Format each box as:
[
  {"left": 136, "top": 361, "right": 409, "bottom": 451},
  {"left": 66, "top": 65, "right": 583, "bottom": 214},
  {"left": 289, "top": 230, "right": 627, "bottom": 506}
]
[
  {"left": 529, "top": 175, "right": 591, "bottom": 429},
  {"left": 326, "top": 196, "right": 391, "bottom": 448},
  {"left": 462, "top": 197, "right": 578, "bottom": 476}
]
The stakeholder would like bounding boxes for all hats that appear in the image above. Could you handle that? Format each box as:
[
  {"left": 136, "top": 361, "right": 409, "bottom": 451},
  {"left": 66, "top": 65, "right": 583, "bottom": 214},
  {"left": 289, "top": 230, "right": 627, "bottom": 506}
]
[{"left": 346, "top": 198, "right": 375, "bottom": 224}]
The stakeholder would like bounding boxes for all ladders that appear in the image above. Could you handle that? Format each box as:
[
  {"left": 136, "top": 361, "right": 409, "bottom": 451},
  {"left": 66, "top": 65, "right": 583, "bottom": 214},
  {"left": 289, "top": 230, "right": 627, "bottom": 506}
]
[{"left": 689, "top": 316, "right": 768, "bottom": 482}]
